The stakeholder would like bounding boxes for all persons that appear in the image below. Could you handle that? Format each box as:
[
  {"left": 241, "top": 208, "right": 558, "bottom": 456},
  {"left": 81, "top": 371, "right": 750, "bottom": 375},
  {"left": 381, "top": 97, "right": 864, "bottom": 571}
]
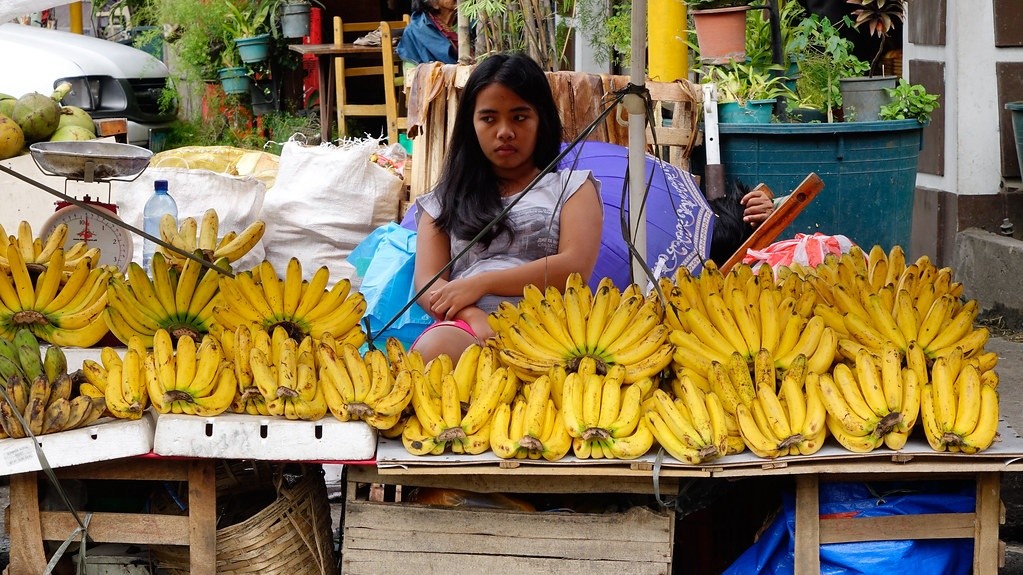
[
  {"left": 408, "top": 52, "right": 605, "bottom": 371},
  {"left": 708, "top": 178, "right": 791, "bottom": 270},
  {"left": 395, "top": 0, "right": 475, "bottom": 109}
]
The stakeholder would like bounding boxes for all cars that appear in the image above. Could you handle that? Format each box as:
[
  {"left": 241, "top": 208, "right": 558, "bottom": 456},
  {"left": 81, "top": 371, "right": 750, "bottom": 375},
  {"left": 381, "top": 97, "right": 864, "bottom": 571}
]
[{"left": 0, "top": 18, "right": 183, "bottom": 149}]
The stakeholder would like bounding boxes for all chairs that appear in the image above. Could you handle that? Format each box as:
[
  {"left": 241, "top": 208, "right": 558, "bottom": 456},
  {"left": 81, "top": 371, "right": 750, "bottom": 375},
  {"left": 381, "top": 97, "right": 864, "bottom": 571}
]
[
  {"left": 333, "top": 15, "right": 408, "bottom": 141},
  {"left": 719, "top": 172, "right": 825, "bottom": 275},
  {"left": 380, "top": 19, "right": 409, "bottom": 148}
]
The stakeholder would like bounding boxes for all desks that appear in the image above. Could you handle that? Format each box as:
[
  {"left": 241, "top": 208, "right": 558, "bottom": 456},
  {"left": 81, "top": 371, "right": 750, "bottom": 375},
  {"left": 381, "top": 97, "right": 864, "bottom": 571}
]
[{"left": 289, "top": 43, "right": 405, "bottom": 145}]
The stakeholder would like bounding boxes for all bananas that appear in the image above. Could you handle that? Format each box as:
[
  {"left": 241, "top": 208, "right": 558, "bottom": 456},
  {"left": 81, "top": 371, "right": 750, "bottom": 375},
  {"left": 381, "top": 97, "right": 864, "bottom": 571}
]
[{"left": 0, "top": 208, "right": 999, "bottom": 459}]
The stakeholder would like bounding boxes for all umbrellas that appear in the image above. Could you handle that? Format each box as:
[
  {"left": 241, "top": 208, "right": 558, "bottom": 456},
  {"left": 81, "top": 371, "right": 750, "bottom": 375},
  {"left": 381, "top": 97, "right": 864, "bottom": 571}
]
[{"left": 398, "top": 140, "right": 721, "bottom": 293}]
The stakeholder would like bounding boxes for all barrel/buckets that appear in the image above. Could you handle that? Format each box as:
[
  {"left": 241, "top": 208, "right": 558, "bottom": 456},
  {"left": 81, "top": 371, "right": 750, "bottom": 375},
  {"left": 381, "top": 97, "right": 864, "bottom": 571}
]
[{"left": 1006, "top": 102, "right": 1022, "bottom": 179}]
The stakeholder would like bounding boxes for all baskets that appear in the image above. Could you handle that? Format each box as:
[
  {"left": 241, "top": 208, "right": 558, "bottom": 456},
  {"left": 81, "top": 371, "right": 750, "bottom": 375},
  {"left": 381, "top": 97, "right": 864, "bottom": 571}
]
[{"left": 152, "top": 458, "right": 333, "bottom": 575}]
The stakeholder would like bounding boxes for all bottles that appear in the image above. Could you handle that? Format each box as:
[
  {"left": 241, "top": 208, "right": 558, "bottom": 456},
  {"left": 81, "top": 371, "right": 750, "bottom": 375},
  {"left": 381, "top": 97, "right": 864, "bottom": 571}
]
[{"left": 143, "top": 180, "right": 178, "bottom": 279}]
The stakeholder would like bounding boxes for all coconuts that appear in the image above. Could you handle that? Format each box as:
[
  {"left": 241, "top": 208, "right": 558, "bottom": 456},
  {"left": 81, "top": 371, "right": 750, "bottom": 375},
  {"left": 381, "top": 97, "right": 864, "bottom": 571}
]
[{"left": 0, "top": 92, "right": 98, "bottom": 160}]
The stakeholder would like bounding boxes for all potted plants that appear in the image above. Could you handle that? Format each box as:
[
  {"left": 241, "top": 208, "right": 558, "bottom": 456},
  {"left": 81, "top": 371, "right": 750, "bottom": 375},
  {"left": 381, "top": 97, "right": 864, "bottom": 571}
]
[
  {"left": 837, "top": 0, "right": 906, "bottom": 121},
  {"left": 663, "top": 0, "right": 929, "bottom": 264},
  {"left": 262, "top": 0, "right": 325, "bottom": 38},
  {"left": 746, "top": 10, "right": 819, "bottom": 89},
  {"left": 693, "top": 49, "right": 801, "bottom": 125},
  {"left": 217, "top": 31, "right": 251, "bottom": 92},
  {"left": 220, "top": 0, "right": 271, "bottom": 62},
  {"left": 682, "top": 0, "right": 751, "bottom": 63}
]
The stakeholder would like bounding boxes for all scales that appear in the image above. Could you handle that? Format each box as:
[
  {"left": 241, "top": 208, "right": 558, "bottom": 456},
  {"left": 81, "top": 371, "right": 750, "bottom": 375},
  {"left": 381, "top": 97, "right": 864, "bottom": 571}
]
[{"left": 30, "top": 140, "right": 157, "bottom": 285}]
